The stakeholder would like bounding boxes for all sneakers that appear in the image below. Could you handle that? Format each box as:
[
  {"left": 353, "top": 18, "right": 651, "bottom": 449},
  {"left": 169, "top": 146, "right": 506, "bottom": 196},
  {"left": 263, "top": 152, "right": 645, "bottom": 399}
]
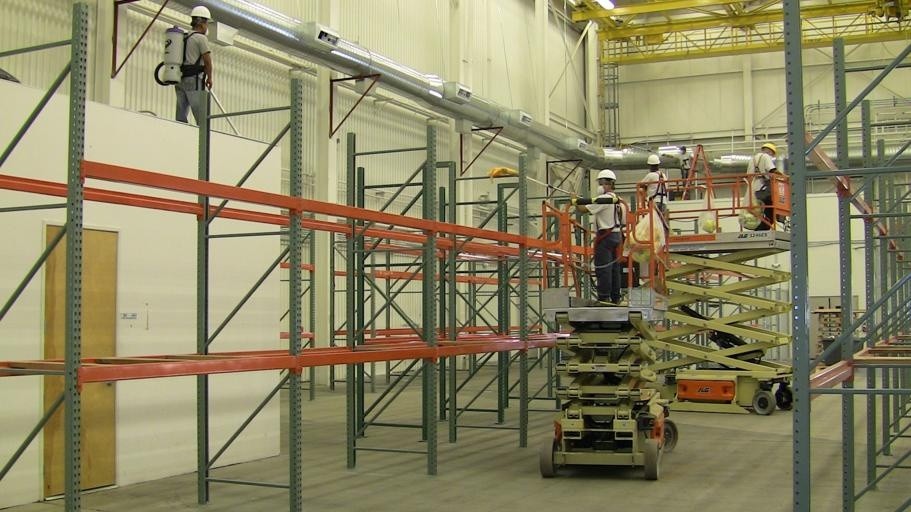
[{"left": 595, "top": 299, "right": 622, "bottom": 306}]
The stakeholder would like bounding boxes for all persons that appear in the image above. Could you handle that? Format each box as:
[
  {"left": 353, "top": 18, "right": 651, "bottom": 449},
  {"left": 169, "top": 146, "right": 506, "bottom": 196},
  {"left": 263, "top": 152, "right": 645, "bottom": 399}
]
[
  {"left": 746, "top": 142, "right": 791, "bottom": 230},
  {"left": 637, "top": 154, "right": 670, "bottom": 238},
  {"left": 174, "top": 5, "right": 214, "bottom": 127},
  {"left": 674, "top": 146, "right": 696, "bottom": 188},
  {"left": 569, "top": 169, "right": 629, "bottom": 306}
]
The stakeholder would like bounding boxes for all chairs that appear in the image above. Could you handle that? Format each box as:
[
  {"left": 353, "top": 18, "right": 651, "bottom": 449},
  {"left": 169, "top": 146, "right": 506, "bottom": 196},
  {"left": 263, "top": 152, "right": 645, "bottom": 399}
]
[{"left": 620, "top": 260, "right": 640, "bottom": 288}]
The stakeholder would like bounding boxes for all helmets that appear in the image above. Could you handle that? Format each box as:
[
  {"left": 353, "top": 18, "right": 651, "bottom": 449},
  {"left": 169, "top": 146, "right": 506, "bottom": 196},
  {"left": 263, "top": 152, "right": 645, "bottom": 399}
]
[
  {"left": 191, "top": 6, "right": 211, "bottom": 19},
  {"left": 647, "top": 155, "right": 661, "bottom": 165},
  {"left": 596, "top": 169, "right": 617, "bottom": 180},
  {"left": 761, "top": 143, "right": 776, "bottom": 154}
]
